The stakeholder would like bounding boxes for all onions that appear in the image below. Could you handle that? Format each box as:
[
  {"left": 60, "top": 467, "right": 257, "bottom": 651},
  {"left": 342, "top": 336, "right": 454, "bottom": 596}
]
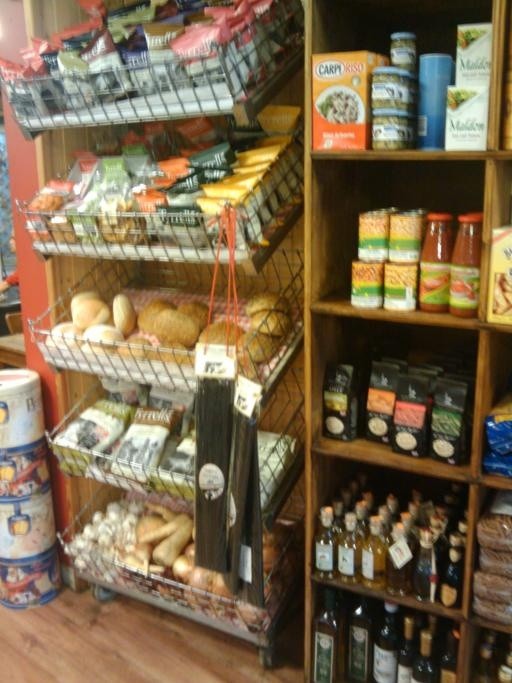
[{"left": 166, "top": 520, "right": 299, "bottom": 601}]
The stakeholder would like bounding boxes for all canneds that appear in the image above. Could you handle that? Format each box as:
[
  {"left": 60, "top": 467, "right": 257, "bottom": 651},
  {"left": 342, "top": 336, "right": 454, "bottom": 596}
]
[
  {"left": 358, "top": 211, "right": 389, "bottom": 262},
  {"left": 386, "top": 211, "right": 424, "bottom": 263},
  {"left": 392, "top": 32, "right": 417, "bottom": 71},
  {"left": 351, "top": 261, "right": 383, "bottom": 307},
  {"left": 370, "top": 113, "right": 415, "bottom": 149},
  {"left": 370, "top": 67, "right": 419, "bottom": 117},
  {"left": 381, "top": 263, "right": 421, "bottom": 309}
]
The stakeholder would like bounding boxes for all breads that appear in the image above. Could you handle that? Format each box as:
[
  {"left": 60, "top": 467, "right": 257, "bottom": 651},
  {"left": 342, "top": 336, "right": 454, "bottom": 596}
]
[{"left": 45, "top": 291, "right": 289, "bottom": 379}]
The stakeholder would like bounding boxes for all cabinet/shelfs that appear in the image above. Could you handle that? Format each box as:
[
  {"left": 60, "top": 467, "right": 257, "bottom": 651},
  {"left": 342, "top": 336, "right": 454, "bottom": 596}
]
[
  {"left": 0, "top": 0, "right": 307, "bottom": 671},
  {"left": 308, "top": 0, "right": 512, "bottom": 683}
]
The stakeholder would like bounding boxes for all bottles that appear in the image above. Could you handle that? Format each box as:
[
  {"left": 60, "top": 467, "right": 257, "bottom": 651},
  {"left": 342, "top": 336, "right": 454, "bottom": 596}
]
[
  {"left": 438, "top": 504, "right": 452, "bottom": 535},
  {"left": 419, "top": 53, "right": 452, "bottom": 150},
  {"left": 373, "top": 601, "right": 398, "bottom": 683},
  {"left": 396, "top": 615, "right": 415, "bottom": 683},
  {"left": 312, "top": 590, "right": 343, "bottom": 683},
  {"left": 315, "top": 507, "right": 338, "bottom": 579},
  {"left": 360, "top": 516, "right": 385, "bottom": 590},
  {"left": 457, "top": 520, "right": 466, "bottom": 539},
  {"left": 453, "top": 484, "right": 463, "bottom": 502},
  {"left": 400, "top": 512, "right": 417, "bottom": 551},
  {"left": 430, "top": 515, "right": 447, "bottom": 553},
  {"left": 413, "top": 489, "right": 424, "bottom": 504},
  {"left": 362, "top": 491, "right": 377, "bottom": 514},
  {"left": 413, "top": 528, "right": 435, "bottom": 600},
  {"left": 379, "top": 504, "right": 392, "bottom": 530},
  {"left": 386, "top": 523, "right": 412, "bottom": 595},
  {"left": 445, "top": 494, "right": 456, "bottom": 522},
  {"left": 439, "top": 534, "right": 461, "bottom": 607},
  {"left": 341, "top": 489, "right": 351, "bottom": 511},
  {"left": 496, "top": 664, "right": 512, "bottom": 683},
  {"left": 332, "top": 499, "right": 343, "bottom": 529},
  {"left": 427, "top": 614, "right": 438, "bottom": 632},
  {"left": 411, "top": 630, "right": 438, "bottom": 683},
  {"left": 439, "top": 630, "right": 458, "bottom": 683},
  {"left": 477, "top": 634, "right": 494, "bottom": 673},
  {"left": 347, "top": 594, "right": 373, "bottom": 683},
  {"left": 409, "top": 502, "right": 420, "bottom": 526},
  {"left": 353, "top": 501, "right": 369, "bottom": 533},
  {"left": 351, "top": 481, "right": 358, "bottom": 497},
  {"left": 338, "top": 513, "right": 360, "bottom": 584},
  {"left": 387, "top": 494, "right": 400, "bottom": 522}
]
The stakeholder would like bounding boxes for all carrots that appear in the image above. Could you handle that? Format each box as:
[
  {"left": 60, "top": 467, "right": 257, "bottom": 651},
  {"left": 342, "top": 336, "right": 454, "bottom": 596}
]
[{"left": 141, "top": 500, "right": 194, "bottom": 565}]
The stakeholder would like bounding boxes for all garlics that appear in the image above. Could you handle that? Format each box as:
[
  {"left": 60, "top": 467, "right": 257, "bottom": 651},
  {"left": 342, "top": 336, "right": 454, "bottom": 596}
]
[{"left": 66, "top": 500, "right": 143, "bottom": 591}]
